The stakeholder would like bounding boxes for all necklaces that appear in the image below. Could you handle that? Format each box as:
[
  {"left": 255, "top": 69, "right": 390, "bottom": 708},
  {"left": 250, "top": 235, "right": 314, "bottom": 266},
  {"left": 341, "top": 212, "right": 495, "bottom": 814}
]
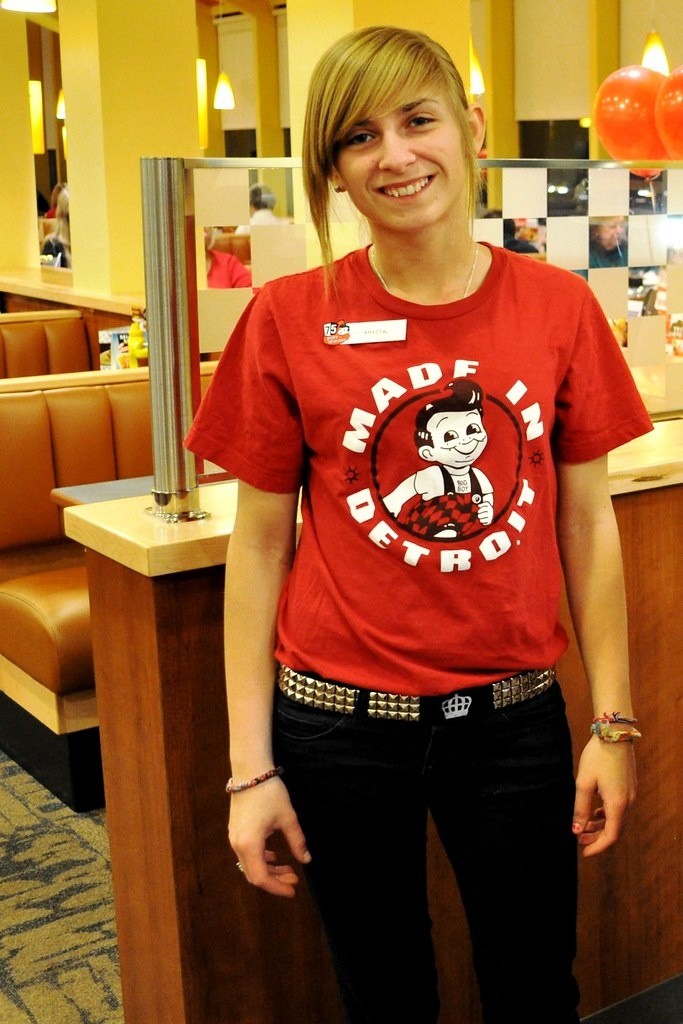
[{"left": 372, "top": 245, "right": 478, "bottom": 298}]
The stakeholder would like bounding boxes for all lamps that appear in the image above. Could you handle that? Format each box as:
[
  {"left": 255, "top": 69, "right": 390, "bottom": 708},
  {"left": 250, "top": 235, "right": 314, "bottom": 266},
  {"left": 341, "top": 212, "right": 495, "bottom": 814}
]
[
  {"left": 213, "top": 0, "right": 236, "bottom": 109},
  {"left": 642, "top": 31, "right": 671, "bottom": 76}
]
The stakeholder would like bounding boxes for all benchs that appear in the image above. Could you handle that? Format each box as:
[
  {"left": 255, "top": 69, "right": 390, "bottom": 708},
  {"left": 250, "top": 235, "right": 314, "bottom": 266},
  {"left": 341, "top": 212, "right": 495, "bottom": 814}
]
[
  {"left": 0, "top": 310, "right": 86, "bottom": 378},
  {"left": 0, "top": 360, "right": 218, "bottom": 814}
]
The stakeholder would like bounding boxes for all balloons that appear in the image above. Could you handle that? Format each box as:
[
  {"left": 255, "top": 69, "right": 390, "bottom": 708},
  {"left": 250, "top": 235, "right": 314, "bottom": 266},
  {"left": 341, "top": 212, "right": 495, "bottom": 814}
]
[
  {"left": 594, "top": 65, "right": 668, "bottom": 180},
  {"left": 655, "top": 65, "right": 683, "bottom": 160}
]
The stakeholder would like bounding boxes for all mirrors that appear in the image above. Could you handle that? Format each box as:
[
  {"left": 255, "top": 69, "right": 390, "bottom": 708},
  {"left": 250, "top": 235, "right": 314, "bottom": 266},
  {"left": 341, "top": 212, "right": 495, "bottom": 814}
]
[
  {"left": 26, "top": 0, "right": 73, "bottom": 289},
  {"left": 198, "top": 0, "right": 292, "bottom": 289}
]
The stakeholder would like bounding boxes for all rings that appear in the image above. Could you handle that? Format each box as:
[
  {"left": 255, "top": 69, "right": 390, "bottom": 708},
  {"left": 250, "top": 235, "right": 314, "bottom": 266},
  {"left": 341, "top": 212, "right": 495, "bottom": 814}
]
[{"left": 236, "top": 862, "right": 243, "bottom": 871}]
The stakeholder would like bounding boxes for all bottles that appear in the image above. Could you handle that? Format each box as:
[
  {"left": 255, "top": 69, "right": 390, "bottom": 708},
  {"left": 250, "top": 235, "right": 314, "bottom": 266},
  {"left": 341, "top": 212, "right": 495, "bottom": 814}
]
[{"left": 128, "top": 307, "right": 149, "bottom": 369}]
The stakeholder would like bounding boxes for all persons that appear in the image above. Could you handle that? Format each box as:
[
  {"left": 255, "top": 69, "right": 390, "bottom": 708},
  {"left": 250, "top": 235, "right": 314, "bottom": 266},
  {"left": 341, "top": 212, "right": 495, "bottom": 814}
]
[
  {"left": 587, "top": 214, "right": 646, "bottom": 288},
  {"left": 236, "top": 185, "right": 284, "bottom": 235},
  {"left": 484, "top": 209, "right": 538, "bottom": 254},
  {"left": 36, "top": 183, "right": 71, "bottom": 267},
  {"left": 204, "top": 228, "right": 253, "bottom": 289},
  {"left": 182, "top": 26, "right": 654, "bottom": 1024}
]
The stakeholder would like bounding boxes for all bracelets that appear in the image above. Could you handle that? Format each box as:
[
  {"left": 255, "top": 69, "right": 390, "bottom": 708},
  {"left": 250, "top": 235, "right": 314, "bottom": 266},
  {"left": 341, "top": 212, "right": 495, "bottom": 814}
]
[
  {"left": 591, "top": 712, "right": 641, "bottom": 742},
  {"left": 226, "top": 767, "right": 283, "bottom": 793}
]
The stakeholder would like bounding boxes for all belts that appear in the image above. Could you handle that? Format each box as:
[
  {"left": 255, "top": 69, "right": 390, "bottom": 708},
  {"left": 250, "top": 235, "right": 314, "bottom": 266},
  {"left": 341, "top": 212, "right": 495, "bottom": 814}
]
[{"left": 274, "top": 663, "right": 557, "bottom": 722}]
